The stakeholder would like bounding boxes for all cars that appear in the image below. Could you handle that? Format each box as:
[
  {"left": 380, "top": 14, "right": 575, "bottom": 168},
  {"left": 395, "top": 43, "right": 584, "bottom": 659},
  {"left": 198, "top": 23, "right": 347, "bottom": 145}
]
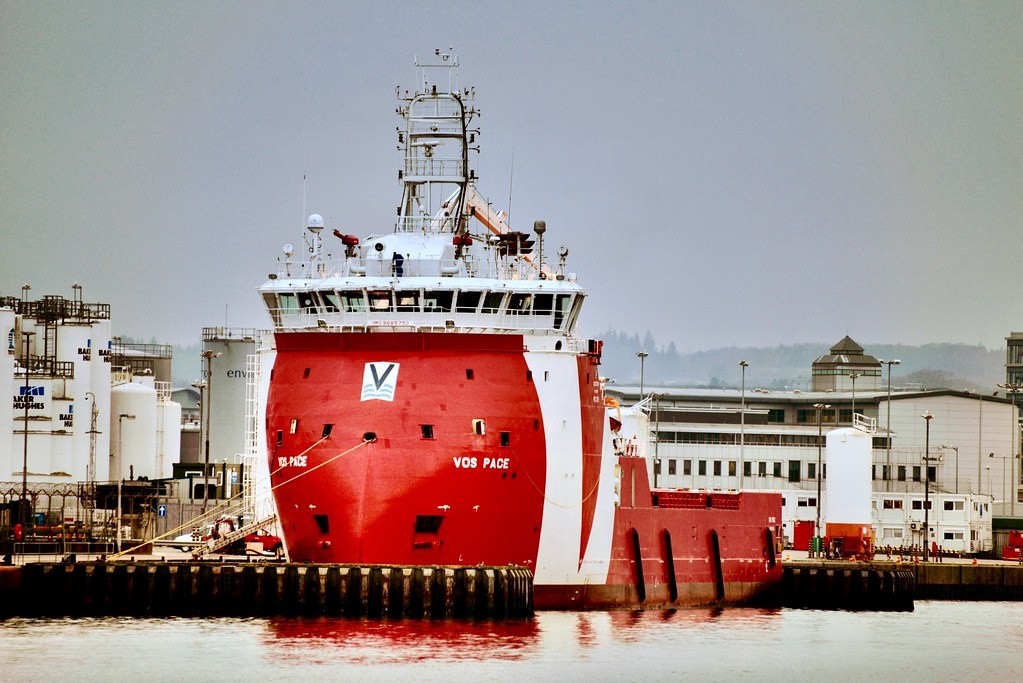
[{"left": 174, "top": 522, "right": 282, "bottom": 553}]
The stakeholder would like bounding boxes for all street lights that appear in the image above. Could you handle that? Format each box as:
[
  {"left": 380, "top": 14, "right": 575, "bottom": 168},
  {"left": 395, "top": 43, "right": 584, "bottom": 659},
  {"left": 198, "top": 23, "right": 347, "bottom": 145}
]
[
  {"left": 953, "top": 446, "right": 958, "bottom": 493},
  {"left": 984, "top": 465, "right": 989, "bottom": 495},
  {"left": 200, "top": 350, "right": 223, "bottom": 520},
  {"left": 878, "top": 359, "right": 900, "bottom": 491},
  {"left": 813, "top": 404, "right": 831, "bottom": 559},
  {"left": 21, "top": 330, "right": 37, "bottom": 541},
  {"left": 190, "top": 378, "right": 205, "bottom": 471},
  {"left": 649, "top": 391, "right": 670, "bottom": 489},
  {"left": 739, "top": 361, "right": 749, "bottom": 491},
  {"left": 117, "top": 413, "right": 136, "bottom": 552},
  {"left": 636, "top": 351, "right": 649, "bottom": 402},
  {"left": 848, "top": 373, "right": 859, "bottom": 426},
  {"left": 85, "top": 391, "right": 98, "bottom": 484},
  {"left": 920, "top": 411, "right": 935, "bottom": 563},
  {"left": 989, "top": 383, "right": 1023, "bottom": 516}
]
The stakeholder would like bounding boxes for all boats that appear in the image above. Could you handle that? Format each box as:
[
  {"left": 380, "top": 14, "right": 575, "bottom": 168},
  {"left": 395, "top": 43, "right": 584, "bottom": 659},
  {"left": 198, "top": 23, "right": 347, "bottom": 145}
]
[
  {"left": 609, "top": 417, "right": 622, "bottom": 432},
  {"left": 253, "top": 45, "right": 785, "bottom": 611}
]
